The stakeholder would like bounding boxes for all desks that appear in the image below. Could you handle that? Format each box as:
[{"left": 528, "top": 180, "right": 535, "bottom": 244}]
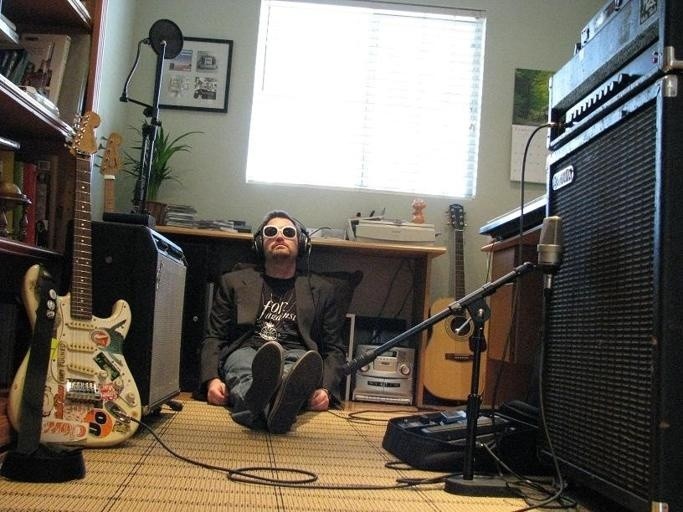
[
  {"left": 150, "top": 221, "right": 449, "bottom": 406},
  {"left": 478, "top": 221, "right": 545, "bottom": 412}
]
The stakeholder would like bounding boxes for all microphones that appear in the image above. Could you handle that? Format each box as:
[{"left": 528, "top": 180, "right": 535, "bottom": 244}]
[{"left": 536, "top": 214, "right": 564, "bottom": 290}]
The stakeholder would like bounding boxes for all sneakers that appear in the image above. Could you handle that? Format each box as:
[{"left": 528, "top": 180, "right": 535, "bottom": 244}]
[
  {"left": 244, "top": 341, "right": 284, "bottom": 416},
  {"left": 267, "top": 350, "right": 323, "bottom": 434}
]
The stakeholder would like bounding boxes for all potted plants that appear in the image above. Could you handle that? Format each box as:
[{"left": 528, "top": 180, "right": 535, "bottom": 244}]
[{"left": 116, "top": 120, "right": 206, "bottom": 223}]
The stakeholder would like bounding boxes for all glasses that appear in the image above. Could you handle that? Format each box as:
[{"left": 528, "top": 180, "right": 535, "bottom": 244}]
[{"left": 262, "top": 225, "right": 298, "bottom": 240}]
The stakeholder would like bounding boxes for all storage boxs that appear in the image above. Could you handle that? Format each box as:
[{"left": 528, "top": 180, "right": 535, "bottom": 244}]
[{"left": 351, "top": 221, "right": 435, "bottom": 249}]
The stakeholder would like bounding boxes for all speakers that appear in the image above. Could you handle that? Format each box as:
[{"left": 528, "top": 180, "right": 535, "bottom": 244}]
[
  {"left": 90, "top": 220, "right": 190, "bottom": 412},
  {"left": 534, "top": 75, "right": 683, "bottom": 512}
]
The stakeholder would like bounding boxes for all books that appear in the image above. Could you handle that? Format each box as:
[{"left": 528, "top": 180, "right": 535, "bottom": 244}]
[
  {"left": 0, "top": 14, "right": 92, "bottom": 129},
  {"left": 1, "top": 151, "right": 57, "bottom": 251},
  {"left": 219, "top": 226, "right": 251, "bottom": 233}
]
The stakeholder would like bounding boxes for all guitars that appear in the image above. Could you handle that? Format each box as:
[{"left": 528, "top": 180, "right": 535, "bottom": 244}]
[
  {"left": 423, "top": 204, "right": 490, "bottom": 401},
  {"left": 8, "top": 111, "right": 141, "bottom": 448}
]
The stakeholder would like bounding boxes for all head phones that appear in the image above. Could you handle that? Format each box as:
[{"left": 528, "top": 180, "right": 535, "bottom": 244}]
[{"left": 253, "top": 218, "right": 312, "bottom": 258}]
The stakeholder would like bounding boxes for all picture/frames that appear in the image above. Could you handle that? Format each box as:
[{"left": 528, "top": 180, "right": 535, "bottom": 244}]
[{"left": 156, "top": 36, "right": 234, "bottom": 114}]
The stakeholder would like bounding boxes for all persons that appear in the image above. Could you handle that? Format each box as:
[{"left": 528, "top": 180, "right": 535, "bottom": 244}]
[{"left": 193, "top": 210, "right": 345, "bottom": 435}]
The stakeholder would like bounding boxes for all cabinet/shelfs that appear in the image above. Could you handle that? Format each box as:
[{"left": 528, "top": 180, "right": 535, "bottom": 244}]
[{"left": 0, "top": 0, "right": 109, "bottom": 449}]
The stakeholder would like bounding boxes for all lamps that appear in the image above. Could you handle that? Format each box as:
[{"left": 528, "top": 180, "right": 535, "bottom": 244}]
[{"left": 102, "top": 20, "right": 183, "bottom": 229}]
